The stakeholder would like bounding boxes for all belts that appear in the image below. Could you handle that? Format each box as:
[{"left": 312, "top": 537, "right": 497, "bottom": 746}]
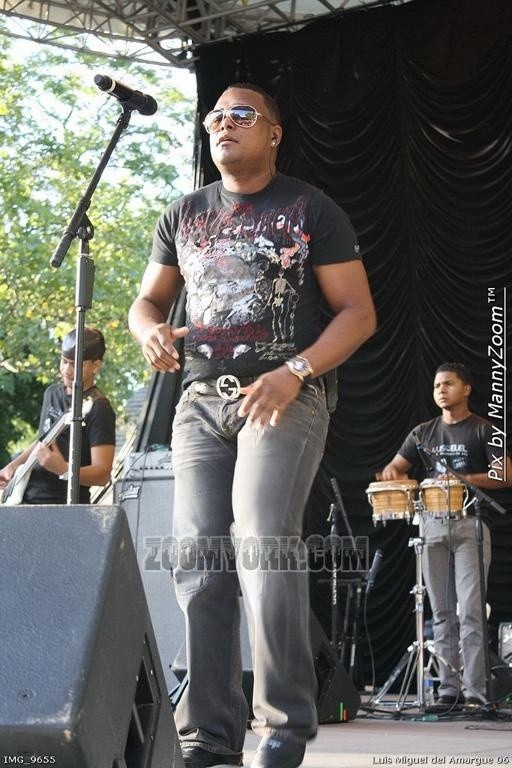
[{"left": 195, "top": 376, "right": 257, "bottom": 401}]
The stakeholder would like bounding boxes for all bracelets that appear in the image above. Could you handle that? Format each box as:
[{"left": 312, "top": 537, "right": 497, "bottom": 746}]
[{"left": 53, "top": 462, "right": 77, "bottom": 485}]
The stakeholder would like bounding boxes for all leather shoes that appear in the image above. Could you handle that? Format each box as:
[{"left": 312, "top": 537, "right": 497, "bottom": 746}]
[
  {"left": 424, "top": 697, "right": 465, "bottom": 714},
  {"left": 462, "top": 697, "right": 488, "bottom": 717}
]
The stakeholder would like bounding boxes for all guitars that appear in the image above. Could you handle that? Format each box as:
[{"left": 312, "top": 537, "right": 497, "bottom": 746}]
[{"left": 0, "top": 396, "right": 95, "bottom": 505}]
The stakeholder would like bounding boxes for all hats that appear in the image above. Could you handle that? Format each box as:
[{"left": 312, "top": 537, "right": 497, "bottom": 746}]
[{"left": 61, "top": 328, "right": 105, "bottom": 360}]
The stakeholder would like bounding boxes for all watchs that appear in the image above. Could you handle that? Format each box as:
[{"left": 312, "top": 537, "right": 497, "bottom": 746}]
[{"left": 284, "top": 353, "right": 316, "bottom": 386}]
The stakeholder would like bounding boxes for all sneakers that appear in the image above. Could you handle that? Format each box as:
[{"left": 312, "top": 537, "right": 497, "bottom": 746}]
[
  {"left": 181, "top": 747, "right": 243, "bottom": 768},
  {"left": 249, "top": 735, "right": 306, "bottom": 768}
]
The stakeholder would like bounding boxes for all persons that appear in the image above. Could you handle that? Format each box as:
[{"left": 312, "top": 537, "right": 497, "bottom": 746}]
[
  {"left": 3, "top": 328, "right": 115, "bottom": 504},
  {"left": 372, "top": 361, "right": 512, "bottom": 716},
  {"left": 128, "top": 77, "right": 380, "bottom": 768}
]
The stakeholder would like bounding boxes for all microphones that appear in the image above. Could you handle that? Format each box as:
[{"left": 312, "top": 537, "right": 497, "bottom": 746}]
[
  {"left": 413, "top": 432, "right": 434, "bottom": 473},
  {"left": 94, "top": 74, "right": 158, "bottom": 116},
  {"left": 364, "top": 548, "right": 383, "bottom": 594}
]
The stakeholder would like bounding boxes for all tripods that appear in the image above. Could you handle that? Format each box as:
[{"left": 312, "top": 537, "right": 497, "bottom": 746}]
[
  {"left": 369, "top": 537, "right": 498, "bottom": 720},
  {"left": 423, "top": 500, "right": 512, "bottom": 723}
]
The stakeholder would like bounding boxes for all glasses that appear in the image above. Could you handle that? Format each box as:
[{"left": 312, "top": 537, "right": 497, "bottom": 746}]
[{"left": 202, "top": 104, "right": 276, "bottom": 135}]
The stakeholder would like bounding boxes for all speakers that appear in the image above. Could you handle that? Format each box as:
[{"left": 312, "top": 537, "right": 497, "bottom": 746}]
[
  {"left": 0, "top": 504, "right": 186, "bottom": 767},
  {"left": 170, "top": 595, "right": 362, "bottom": 730}
]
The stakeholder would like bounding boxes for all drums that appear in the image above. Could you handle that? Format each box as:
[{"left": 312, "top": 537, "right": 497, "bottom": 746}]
[
  {"left": 365, "top": 477, "right": 419, "bottom": 527},
  {"left": 419, "top": 477, "right": 465, "bottom": 521}
]
